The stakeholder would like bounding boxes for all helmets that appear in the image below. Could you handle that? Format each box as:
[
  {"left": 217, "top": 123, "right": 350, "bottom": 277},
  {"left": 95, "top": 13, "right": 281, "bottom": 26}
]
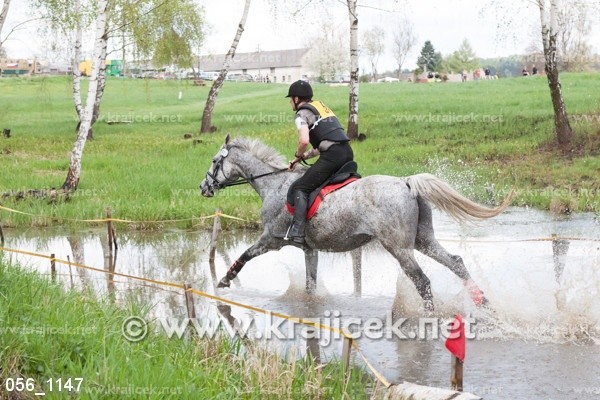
[{"left": 285, "top": 80, "right": 313, "bottom": 110}]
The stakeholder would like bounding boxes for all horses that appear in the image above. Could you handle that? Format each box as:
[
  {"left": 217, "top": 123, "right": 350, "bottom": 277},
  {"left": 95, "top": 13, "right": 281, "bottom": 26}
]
[{"left": 198, "top": 134, "right": 517, "bottom": 314}]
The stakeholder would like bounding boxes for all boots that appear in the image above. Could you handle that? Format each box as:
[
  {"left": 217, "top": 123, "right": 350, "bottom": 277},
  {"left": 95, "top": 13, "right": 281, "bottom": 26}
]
[{"left": 275, "top": 189, "right": 308, "bottom": 244}]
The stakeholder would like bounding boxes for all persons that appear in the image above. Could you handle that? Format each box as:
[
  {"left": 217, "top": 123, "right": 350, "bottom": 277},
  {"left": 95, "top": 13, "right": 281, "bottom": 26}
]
[
  {"left": 424, "top": 62, "right": 539, "bottom": 84},
  {"left": 283, "top": 79, "right": 358, "bottom": 247}
]
